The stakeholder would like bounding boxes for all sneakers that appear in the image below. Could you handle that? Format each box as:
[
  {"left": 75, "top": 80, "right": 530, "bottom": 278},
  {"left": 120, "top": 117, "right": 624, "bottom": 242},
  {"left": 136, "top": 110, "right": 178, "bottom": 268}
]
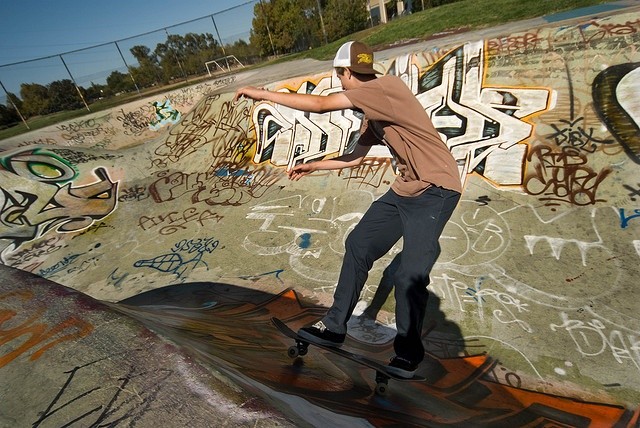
[
  {"left": 387, "top": 354, "right": 421, "bottom": 377},
  {"left": 297, "top": 319, "right": 345, "bottom": 348}
]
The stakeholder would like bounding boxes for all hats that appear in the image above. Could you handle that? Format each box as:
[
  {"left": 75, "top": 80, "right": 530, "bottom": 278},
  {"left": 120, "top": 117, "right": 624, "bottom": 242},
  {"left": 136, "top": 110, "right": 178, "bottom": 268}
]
[{"left": 333, "top": 39, "right": 384, "bottom": 75}]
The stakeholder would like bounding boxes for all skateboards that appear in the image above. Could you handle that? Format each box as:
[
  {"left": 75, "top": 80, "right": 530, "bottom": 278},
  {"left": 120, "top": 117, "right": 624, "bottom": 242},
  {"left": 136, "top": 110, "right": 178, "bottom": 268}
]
[{"left": 270, "top": 317, "right": 426, "bottom": 396}]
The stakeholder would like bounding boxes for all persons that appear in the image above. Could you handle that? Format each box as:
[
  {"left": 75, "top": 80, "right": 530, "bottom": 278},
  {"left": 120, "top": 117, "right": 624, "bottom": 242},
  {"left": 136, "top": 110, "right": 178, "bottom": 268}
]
[{"left": 232, "top": 39, "right": 462, "bottom": 377}]
